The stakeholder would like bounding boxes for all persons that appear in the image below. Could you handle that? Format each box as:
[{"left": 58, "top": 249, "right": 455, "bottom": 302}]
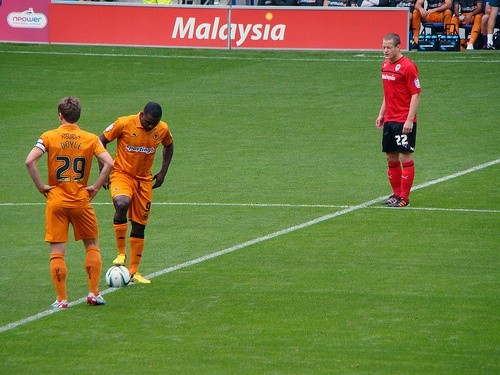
[
  {"left": 376, "top": 32, "right": 422, "bottom": 207},
  {"left": 25, "top": 97, "right": 114, "bottom": 308},
  {"left": 142, "top": 0, "right": 500, "bottom": 50},
  {"left": 97, "top": 101, "right": 173, "bottom": 283}
]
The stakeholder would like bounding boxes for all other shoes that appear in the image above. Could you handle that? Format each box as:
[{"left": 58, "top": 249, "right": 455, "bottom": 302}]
[
  {"left": 410, "top": 41, "right": 420, "bottom": 51},
  {"left": 486, "top": 44, "right": 496, "bottom": 50},
  {"left": 466, "top": 42, "right": 474, "bottom": 50}
]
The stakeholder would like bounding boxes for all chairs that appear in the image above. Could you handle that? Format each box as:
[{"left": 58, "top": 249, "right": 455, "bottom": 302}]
[{"left": 423, "top": 22, "right": 474, "bottom": 39}]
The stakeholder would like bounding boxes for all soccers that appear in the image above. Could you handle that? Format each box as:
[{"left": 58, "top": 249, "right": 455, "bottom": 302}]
[{"left": 105, "top": 266, "right": 130, "bottom": 289}]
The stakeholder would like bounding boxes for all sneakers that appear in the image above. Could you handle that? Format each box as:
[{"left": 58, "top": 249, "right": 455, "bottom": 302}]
[
  {"left": 113, "top": 252, "right": 128, "bottom": 266},
  {"left": 50, "top": 299, "right": 68, "bottom": 308},
  {"left": 388, "top": 198, "right": 410, "bottom": 207},
  {"left": 86, "top": 292, "right": 106, "bottom": 305},
  {"left": 130, "top": 271, "right": 151, "bottom": 284},
  {"left": 381, "top": 195, "right": 398, "bottom": 205}
]
